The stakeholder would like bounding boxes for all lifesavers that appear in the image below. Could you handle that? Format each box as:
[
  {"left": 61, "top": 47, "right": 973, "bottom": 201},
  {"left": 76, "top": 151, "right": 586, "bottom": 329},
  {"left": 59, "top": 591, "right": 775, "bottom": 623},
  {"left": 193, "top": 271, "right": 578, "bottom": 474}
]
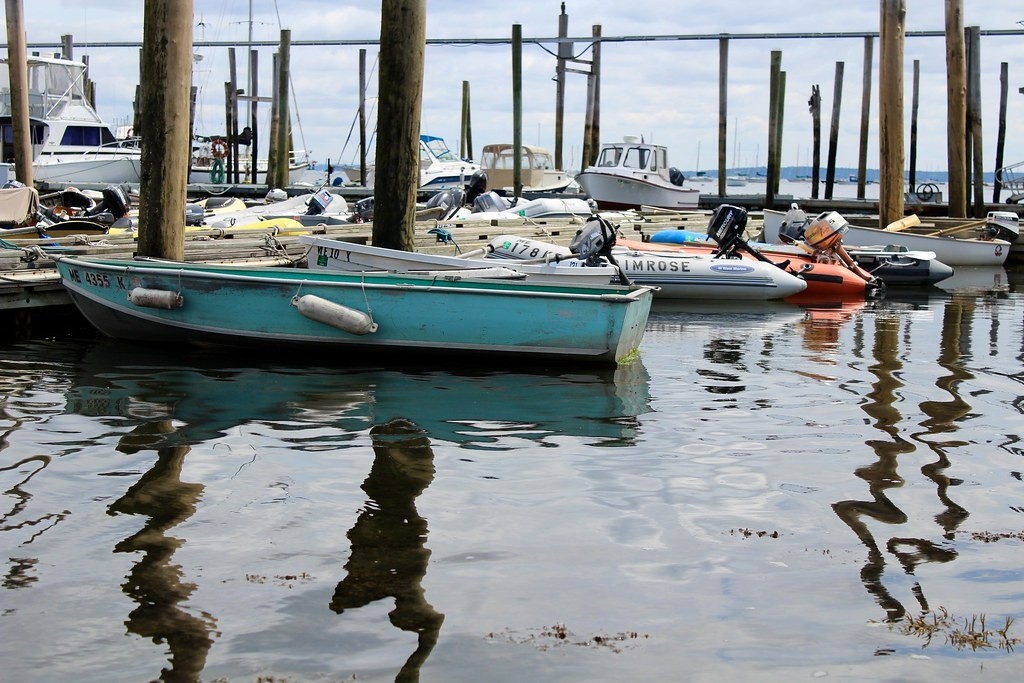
[{"left": 211, "top": 139, "right": 230, "bottom": 158}]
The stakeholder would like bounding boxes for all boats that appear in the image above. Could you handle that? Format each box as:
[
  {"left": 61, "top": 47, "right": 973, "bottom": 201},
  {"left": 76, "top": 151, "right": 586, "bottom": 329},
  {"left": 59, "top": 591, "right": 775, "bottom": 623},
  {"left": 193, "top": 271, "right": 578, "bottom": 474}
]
[
  {"left": 60, "top": 346, "right": 660, "bottom": 451},
  {"left": 0, "top": 0, "right": 1024, "bottom": 313},
  {"left": 47, "top": 244, "right": 663, "bottom": 372}
]
